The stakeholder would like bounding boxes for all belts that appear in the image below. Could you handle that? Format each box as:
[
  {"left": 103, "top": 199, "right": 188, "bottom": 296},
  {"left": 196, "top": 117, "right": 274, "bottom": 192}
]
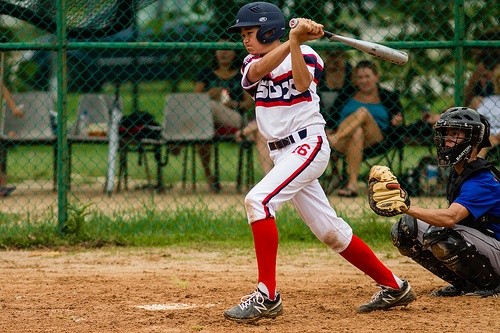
[{"left": 268, "top": 129, "right": 307, "bottom": 151}]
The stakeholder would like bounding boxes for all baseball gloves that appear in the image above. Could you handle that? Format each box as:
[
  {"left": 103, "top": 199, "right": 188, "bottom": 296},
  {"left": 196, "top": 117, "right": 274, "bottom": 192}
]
[{"left": 367, "top": 165, "right": 410, "bottom": 216}]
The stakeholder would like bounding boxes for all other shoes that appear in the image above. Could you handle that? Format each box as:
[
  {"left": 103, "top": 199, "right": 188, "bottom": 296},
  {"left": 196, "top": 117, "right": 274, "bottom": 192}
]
[
  {"left": 475, "top": 284, "right": 500, "bottom": 298},
  {"left": 326, "top": 136, "right": 345, "bottom": 157},
  {"left": 0, "top": 184, "right": 17, "bottom": 196},
  {"left": 337, "top": 188, "right": 358, "bottom": 197},
  {"left": 431, "top": 281, "right": 476, "bottom": 296}
]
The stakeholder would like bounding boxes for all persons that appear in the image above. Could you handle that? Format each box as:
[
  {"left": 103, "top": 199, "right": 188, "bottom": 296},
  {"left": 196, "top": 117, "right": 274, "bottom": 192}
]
[
  {"left": 0, "top": 75, "right": 24, "bottom": 196},
  {"left": 224, "top": 2, "right": 417, "bottom": 321},
  {"left": 235, "top": 119, "right": 274, "bottom": 175},
  {"left": 369, "top": 106, "right": 500, "bottom": 297},
  {"left": 423, "top": 50, "right": 500, "bottom": 163},
  {"left": 325, "top": 60, "right": 406, "bottom": 198},
  {"left": 194, "top": 37, "right": 252, "bottom": 194}
]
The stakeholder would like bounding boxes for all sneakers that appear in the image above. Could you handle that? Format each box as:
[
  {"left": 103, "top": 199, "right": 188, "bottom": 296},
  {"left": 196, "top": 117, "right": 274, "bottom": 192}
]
[
  {"left": 356, "top": 279, "right": 416, "bottom": 314},
  {"left": 222, "top": 287, "right": 283, "bottom": 323}
]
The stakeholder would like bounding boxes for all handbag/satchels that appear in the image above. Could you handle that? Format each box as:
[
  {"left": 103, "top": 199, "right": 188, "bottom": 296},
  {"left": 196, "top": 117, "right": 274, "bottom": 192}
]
[{"left": 119, "top": 110, "right": 162, "bottom": 141}]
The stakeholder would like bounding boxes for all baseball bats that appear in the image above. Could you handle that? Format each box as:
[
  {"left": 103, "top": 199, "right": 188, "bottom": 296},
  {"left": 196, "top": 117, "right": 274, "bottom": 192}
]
[{"left": 289, "top": 17, "right": 408, "bottom": 66}]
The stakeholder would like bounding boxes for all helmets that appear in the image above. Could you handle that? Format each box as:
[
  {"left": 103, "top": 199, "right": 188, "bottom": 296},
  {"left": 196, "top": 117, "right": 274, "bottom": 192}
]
[
  {"left": 432, "top": 107, "right": 492, "bottom": 168},
  {"left": 227, "top": 2, "right": 288, "bottom": 44}
]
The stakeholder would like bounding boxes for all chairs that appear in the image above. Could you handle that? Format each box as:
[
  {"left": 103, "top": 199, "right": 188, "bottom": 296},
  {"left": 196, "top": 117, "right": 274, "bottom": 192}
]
[
  {"left": 160, "top": 92, "right": 255, "bottom": 193},
  {"left": 320, "top": 91, "right": 406, "bottom": 195},
  {"left": 0, "top": 92, "right": 57, "bottom": 189},
  {"left": 67, "top": 95, "right": 123, "bottom": 193}
]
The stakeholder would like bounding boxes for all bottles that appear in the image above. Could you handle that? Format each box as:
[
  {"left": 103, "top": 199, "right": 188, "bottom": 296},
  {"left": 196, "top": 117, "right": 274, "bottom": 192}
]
[{"left": 77, "top": 110, "right": 89, "bottom": 136}]
